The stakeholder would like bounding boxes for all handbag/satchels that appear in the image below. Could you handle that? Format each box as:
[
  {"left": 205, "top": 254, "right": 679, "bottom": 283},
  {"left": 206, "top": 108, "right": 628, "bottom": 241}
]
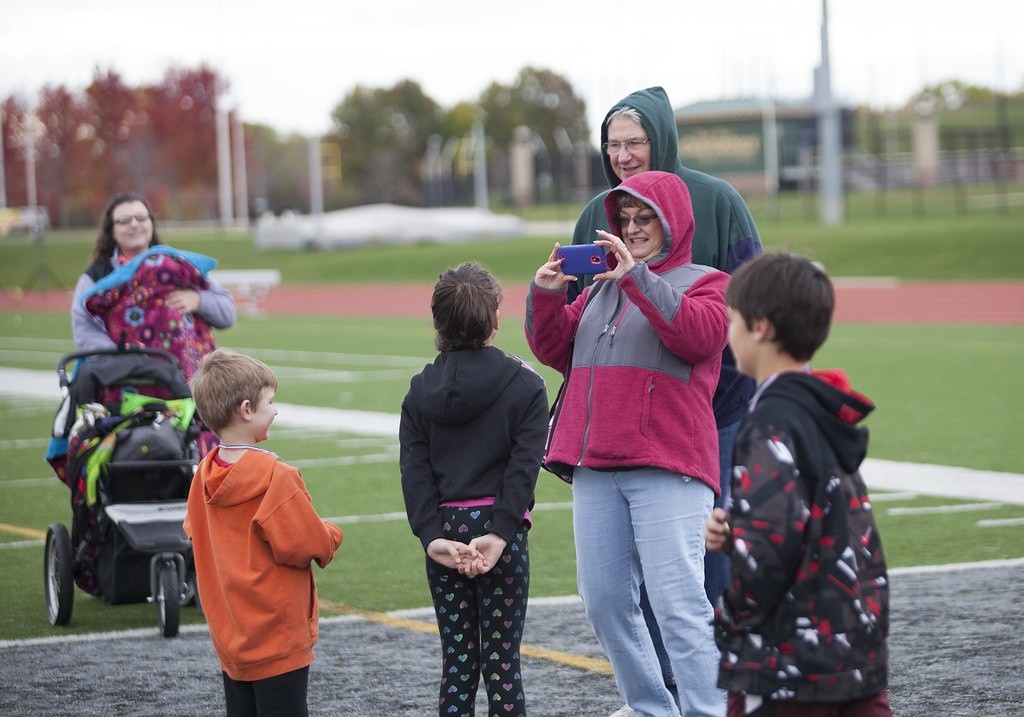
[{"left": 541, "top": 381, "right": 572, "bottom": 484}]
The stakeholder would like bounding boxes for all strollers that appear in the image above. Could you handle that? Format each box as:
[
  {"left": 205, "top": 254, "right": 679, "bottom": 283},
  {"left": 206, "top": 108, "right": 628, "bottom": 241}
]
[{"left": 44, "top": 352, "right": 197, "bottom": 637}]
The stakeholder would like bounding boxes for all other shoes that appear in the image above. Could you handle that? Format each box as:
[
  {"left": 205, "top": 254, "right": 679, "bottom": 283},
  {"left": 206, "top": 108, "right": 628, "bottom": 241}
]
[{"left": 609, "top": 704, "right": 636, "bottom": 717}]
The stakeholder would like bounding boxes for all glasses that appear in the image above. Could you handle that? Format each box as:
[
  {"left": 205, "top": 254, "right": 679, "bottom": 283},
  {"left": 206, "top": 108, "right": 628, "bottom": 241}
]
[
  {"left": 615, "top": 214, "right": 658, "bottom": 227},
  {"left": 113, "top": 214, "right": 150, "bottom": 224},
  {"left": 603, "top": 138, "right": 650, "bottom": 155}
]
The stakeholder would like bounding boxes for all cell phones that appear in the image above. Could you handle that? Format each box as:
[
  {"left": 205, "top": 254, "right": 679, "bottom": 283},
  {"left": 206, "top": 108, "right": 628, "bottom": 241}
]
[{"left": 557, "top": 244, "right": 607, "bottom": 275}]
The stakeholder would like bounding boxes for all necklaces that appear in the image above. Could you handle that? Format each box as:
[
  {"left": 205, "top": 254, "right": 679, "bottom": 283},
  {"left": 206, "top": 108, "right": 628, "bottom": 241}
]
[{"left": 219, "top": 444, "right": 267, "bottom": 452}]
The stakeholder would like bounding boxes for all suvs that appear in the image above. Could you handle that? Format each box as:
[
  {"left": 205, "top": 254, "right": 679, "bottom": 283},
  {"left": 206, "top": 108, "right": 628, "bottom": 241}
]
[{"left": 13, "top": 206, "right": 51, "bottom": 236}]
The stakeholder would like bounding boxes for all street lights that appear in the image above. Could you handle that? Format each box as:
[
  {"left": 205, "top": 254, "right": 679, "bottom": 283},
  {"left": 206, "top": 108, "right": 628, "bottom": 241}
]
[
  {"left": 215, "top": 93, "right": 490, "bottom": 227},
  {"left": 0, "top": 111, "right": 7, "bottom": 206},
  {"left": 25, "top": 85, "right": 39, "bottom": 206}
]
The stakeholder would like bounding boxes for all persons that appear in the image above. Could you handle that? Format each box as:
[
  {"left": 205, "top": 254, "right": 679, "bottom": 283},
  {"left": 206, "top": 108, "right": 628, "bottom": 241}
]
[
  {"left": 398, "top": 260, "right": 549, "bottom": 717},
  {"left": 705, "top": 250, "right": 892, "bottom": 717},
  {"left": 526, "top": 172, "right": 732, "bottom": 717},
  {"left": 72, "top": 191, "right": 236, "bottom": 465},
  {"left": 568, "top": 88, "right": 769, "bottom": 717},
  {"left": 182, "top": 349, "right": 344, "bottom": 717}
]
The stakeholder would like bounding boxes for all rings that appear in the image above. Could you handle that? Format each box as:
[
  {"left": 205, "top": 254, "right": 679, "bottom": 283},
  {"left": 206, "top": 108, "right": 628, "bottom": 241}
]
[{"left": 624, "top": 248, "right": 627, "bottom": 251}]
